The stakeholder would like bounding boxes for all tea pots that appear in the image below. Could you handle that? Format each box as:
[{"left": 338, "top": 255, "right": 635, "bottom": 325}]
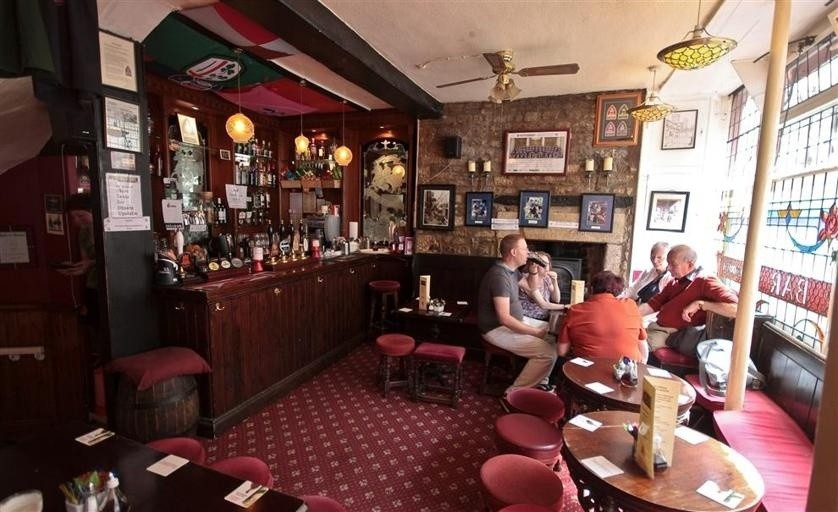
[
  {"left": 621, "top": 360, "right": 639, "bottom": 388},
  {"left": 433, "top": 298, "right": 446, "bottom": 312}
]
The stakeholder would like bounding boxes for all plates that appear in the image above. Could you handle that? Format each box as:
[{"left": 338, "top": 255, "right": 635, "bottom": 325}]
[
  {"left": 221, "top": 260, "right": 231, "bottom": 269},
  {"left": 209, "top": 262, "right": 219, "bottom": 271},
  {"left": 231, "top": 258, "right": 243, "bottom": 268}
]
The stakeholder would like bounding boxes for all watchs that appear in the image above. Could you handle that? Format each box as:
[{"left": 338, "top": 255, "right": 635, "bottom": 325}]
[
  {"left": 697, "top": 300, "right": 704, "bottom": 311},
  {"left": 563, "top": 303, "right": 568, "bottom": 312}
]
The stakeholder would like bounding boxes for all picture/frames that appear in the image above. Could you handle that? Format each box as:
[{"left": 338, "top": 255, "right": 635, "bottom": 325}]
[
  {"left": 103, "top": 93, "right": 143, "bottom": 154},
  {"left": 517, "top": 190, "right": 551, "bottom": 228},
  {"left": 417, "top": 184, "right": 456, "bottom": 231},
  {"left": 465, "top": 191, "right": 493, "bottom": 226},
  {"left": 661, "top": 109, "right": 698, "bottom": 150},
  {"left": 593, "top": 92, "right": 641, "bottom": 146},
  {"left": 578, "top": 192, "right": 616, "bottom": 233},
  {"left": 646, "top": 190, "right": 690, "bottom": 232},
  {"left": 501, "top": 128, "right": 572, "bottom": 177},
  {"left": 176, "top": 112, "right": 200, "bottom": 145}
]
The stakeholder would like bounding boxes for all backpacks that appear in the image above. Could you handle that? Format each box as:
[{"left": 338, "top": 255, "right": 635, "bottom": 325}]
[{"left": 696, "top": 339, "right": 768, "bottom": 397}]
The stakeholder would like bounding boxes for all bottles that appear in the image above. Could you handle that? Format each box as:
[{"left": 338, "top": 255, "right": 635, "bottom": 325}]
[
  {"left": 176, "top": 228, "right": 184, "bottom": 255},
  {"left": 260, "top": 190, "right": 266, "bottom": 208},
  {"left": 280, "top": 220, "right": 285, "bottom": 241},
  {"left": 299, "top": 220, "right": 305, "bottom": 243},
  {"left": 319, "top": 141, "right": 325, "bottom": 161},
  {"left": 267, "top": 220, "right": 274, "bottom": 250},
  {"left": 265, "top": 191, "right": 271, "bottom": 208},
  {"left": 235, "top": 161, "right": 276, "bottom": 188},
  {"left": 289, "top": 219, "right": 294, "bottom": 242},
  {"left": 235, "top": 139, "right": 273, "bottom": 158},
  {"left": 310, "top": 138, "right": 317, "bottom": 161},
  {"left": 215, "top": 198, "right": 227, "bottom": 224}
]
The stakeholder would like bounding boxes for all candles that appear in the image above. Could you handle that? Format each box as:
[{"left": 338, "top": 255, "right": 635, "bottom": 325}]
[
  {"left": 604, "top": 158, "right": 612, "bottom": 170},
  {"left": 468, "top": 160, "right": 475, "bottom": 172},
  {"left": 586, "top": 159, "right": 594, "bottom": 171},
  {"left": 483, "top": 160, "right": 490, "bottom": 171}
]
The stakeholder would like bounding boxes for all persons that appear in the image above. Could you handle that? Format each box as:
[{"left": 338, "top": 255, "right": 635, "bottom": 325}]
[
  {"left": 616, "top": 242, "right": 675, "bottom": 309},
  {"left": 476, "top": 234, "right": 558, "bottom": 414},
  {"left": 640, "top": 244, "right": 739, "bottom": 368},
  {"left": 518, "top": 250, "right": 574, "bottom": 320},
  {"left": 556, "top": 270, "right": 649, "bottom": 419}
]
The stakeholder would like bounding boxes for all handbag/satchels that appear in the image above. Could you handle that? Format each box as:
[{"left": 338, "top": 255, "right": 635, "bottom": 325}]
[{"left": 665, "top": 325, "right": 707, "bottom": 357}]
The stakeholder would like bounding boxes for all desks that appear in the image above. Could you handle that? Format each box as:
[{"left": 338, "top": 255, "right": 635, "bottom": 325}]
[{"left": 1, "top": 410, "right": 303, "bottom": 512}]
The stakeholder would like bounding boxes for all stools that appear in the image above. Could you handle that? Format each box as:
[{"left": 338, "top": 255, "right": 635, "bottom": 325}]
[
  {"left": 206, "top": 456, "right": 273, "bottom": 493},
  {"left": 505, "top": 388, "right": 565, "bottom": 428},
  {"left": 686, "top": 374, "right": 785, "bottom": 431},
  {"left": 495, "top": 414, "right": 563, "bottom": 472},
  {"left": 370, "top": 281, "right": 400, "bottom": 339},
  {"left": 377, "top": 334, "right": 415, "bottom": 397},
  {"left": 145, "top": 438, "right": 205, "bottom": 465},
  {"left": 297, "top": 495, "right": 346, "bottom": 512},
  {"left": 414, "top": 342, "right": 467, "bottom": 407},
  {"left": 479, "top": 454, "right": 563, "bottom": 512},
  {"left": 499, "top": 503, "right": 557, "bottom": 512},
  {"left": 654, "top": 348, "right": 696, "bottom": 370},
  {"left": 479, "top": 338, "right": 518, "bottom": 397}
]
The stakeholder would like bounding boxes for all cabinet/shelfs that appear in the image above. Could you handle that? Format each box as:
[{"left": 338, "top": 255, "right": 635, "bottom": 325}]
[{"left": 235, "top": 154, "right": 279, "bottom": 226}]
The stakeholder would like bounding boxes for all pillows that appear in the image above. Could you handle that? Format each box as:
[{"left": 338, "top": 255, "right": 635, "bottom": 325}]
[{"left": 108, "top": 346, "right": 211, "bottom": 390}]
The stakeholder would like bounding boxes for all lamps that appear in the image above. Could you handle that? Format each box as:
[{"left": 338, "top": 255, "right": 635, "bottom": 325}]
[
  {"left": 468, "top": 158, "right": 491, "bottom": 186},
  {"left": 630, "top": 66, "right": 675, "bottom": 122},
  {"left": 584, "top": 152, "right": 612, "bottom": 186},
  {"left": 225, "top": 49, "right": 255, "bottom": 143},
  {"left": 334, "top": 101, "right": 353, "bottom": 166},
  {"left": 487, "top": 74, "right": 521, "bottom": 104},
  {"left": 295, "top": 80, "right": 309, "bottom": 153},
  {"left": 657, "top": 0, "right": 738, "bottom": 71}
]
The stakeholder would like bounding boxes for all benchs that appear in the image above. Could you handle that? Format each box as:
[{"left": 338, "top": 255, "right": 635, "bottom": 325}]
[{"left": 714, "top": 411, "right": 813, "bottom": 512}]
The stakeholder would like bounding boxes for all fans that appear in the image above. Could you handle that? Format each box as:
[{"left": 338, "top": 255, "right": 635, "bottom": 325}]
[{"left": 436, "top": 51, "right": 579, "bottom": 88}]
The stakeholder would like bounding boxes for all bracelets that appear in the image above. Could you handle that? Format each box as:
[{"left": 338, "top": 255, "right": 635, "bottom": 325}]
[{"left": 528, "top": 272, "right": 539, "bottom": 275}]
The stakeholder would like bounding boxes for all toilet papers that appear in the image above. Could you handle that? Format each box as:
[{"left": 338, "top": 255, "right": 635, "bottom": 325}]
[{"left": 349, "top": 222, "right": 358, "bottom": 240}]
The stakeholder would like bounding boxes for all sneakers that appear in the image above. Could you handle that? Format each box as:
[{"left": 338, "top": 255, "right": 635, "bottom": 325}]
[
  {"left": 498, "top": 395, "right": 522, "bottom": 413},
  {"left": 531, "top": 382, "right": 555, "bottom": 394}
]
